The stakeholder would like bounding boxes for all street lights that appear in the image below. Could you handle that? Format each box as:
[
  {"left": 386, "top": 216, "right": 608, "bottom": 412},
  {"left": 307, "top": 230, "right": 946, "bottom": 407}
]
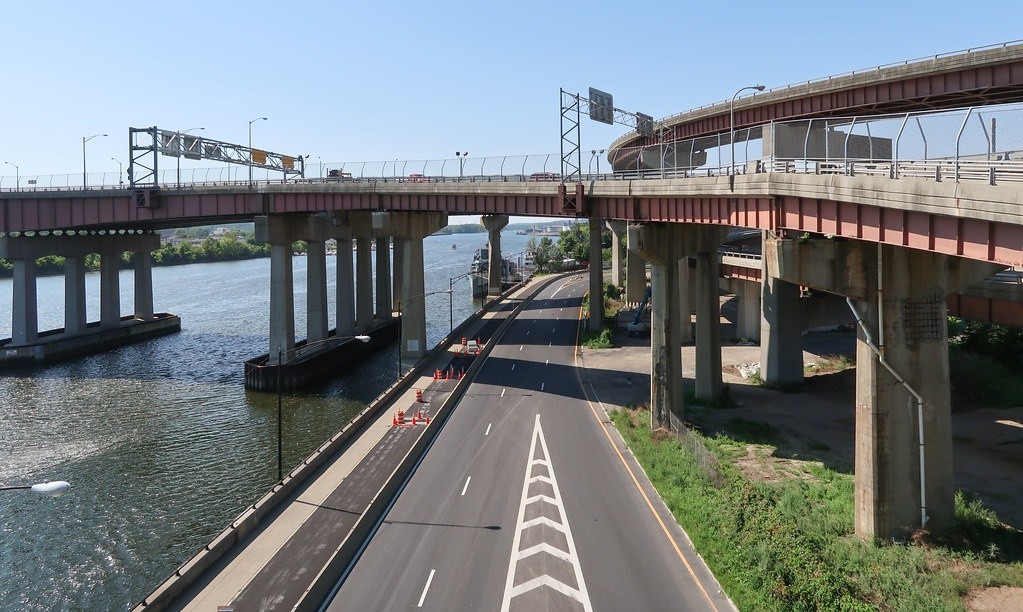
[
  {"left": 248, "top": 117, "right": 269, "bottom": 187},
  {"left": 4, "top": 160, "right": 19, "bottom": 193},
  {"left": 277, "top": 335, "right": 372, "bottom": 482},
  {"left": 729, "top": 83, "right": 766, "bottom": 177},
  {"left": 397, "top": 288, "right": 454, "bottom": 379},
  {"left": 455, "top": 150, "right": 470, "bottom": 183},
  {"left": 111, "top": 158, "right": 122, "bottom": 191},
  {"left": 82, "top": 133, "right": 108, "bottom": 189},
  {"left": 175, "top": 126, "right": 205, "bottom": 187}
]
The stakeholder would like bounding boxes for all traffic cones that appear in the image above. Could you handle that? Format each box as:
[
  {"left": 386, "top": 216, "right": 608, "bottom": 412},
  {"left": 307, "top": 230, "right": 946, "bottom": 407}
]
[
  {"left": 460, "top": 368, "right": 465, "bottom": 377},
  {"left": 426, "top": 413, "right": 430, "bottom": 425},
  {"left": 455, "top": 347, "right": 476, "bottom": 359},
  {"left": 393, "top": 413, "right": 396, "bottom": 425},
  {"left": 433, "top": 371, "right": 437, "bottom": 380},
  {"left": 445, "top": 370, "right": 450, "bottom": 381},
  {"left": 417, "top": 408, "right": 423, "bottom": 420},
  {"left": 458, "top": 372, "right": 461, "bottom": 380},
  {"left": 410, "top": 413, "right": 417, "bottom": 425},
  {"left": 451, "top": 367, "right": 455, "bottom": 379}
]
